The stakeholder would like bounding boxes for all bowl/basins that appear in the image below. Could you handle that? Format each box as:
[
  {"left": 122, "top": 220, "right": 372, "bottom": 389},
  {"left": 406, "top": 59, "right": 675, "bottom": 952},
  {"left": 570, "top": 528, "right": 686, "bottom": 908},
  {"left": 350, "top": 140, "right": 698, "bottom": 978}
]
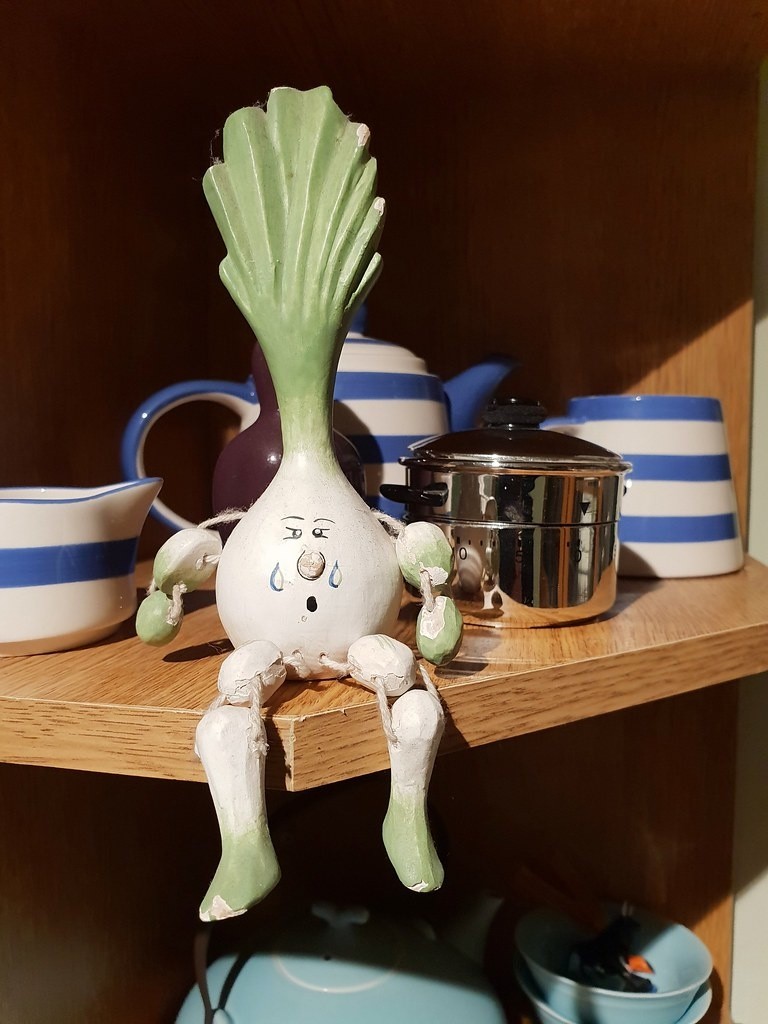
[{"left": 511, "top": 901, "right": 717, "bottom": 1024}]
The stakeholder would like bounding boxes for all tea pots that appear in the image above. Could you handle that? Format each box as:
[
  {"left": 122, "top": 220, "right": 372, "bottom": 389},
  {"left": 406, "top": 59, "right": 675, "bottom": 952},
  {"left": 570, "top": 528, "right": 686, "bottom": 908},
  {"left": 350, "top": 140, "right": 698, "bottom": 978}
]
[{"left": 173, "top": 773, "right": 510, "bottom": 1024}]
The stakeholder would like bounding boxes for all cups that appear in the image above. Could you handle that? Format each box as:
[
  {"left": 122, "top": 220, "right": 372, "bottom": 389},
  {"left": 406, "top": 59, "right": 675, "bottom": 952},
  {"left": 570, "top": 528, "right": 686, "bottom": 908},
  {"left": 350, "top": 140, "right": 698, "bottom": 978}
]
[
  {"left": 538, "top": 394, "right": 745, "bottom": 579},
  {"left": 0, "top": 477, "right": 164, "bottom": 658}
]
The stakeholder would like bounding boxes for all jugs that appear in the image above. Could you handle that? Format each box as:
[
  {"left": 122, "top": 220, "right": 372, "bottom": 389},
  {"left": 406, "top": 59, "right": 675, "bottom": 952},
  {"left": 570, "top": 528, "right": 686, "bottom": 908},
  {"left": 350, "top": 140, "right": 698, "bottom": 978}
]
[{"left": 121, "top": 305, "right": 512, "bottom": 540}]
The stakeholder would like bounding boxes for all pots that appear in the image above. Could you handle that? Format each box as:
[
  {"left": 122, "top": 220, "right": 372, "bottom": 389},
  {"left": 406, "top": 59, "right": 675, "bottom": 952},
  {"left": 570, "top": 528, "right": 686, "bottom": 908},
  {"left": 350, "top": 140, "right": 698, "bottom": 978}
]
[{"left": 379, "top": 397, "right": 633, "bottom": 630}]
